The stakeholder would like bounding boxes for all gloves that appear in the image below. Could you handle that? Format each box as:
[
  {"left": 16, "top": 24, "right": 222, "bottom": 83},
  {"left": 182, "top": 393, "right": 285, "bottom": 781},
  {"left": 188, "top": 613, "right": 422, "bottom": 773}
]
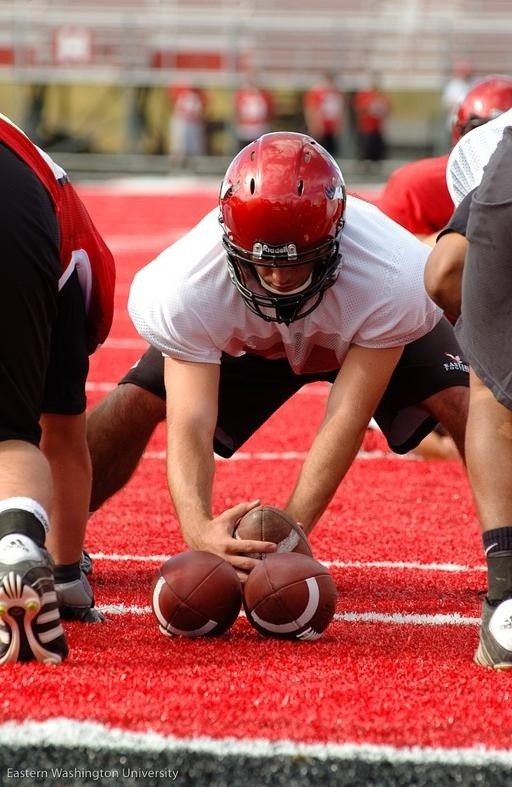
[{"left": 52, "top": 562, "right": 105, "bottom": 623}]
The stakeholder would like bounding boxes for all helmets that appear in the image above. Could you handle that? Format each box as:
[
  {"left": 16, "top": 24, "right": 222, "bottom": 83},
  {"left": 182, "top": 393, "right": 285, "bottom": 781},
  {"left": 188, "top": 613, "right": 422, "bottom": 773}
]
[
  {"left": 215, "top": 131, "right": 347, "bottom": 327},
  {"left": 452, "top": 78, "right": 512, "bottom": 151}
]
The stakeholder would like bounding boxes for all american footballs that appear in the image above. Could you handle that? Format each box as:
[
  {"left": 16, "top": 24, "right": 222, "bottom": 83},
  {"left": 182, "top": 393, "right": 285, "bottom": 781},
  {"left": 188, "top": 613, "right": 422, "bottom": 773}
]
[
  {"left": 230, "top": 505, "right": 314, "bottom": 578},
  {"left": 242, "top": 551, "right": 338, "bottom": 643},
  {"left": 150, "top": 550, "right": 242, "bottom": 641}
]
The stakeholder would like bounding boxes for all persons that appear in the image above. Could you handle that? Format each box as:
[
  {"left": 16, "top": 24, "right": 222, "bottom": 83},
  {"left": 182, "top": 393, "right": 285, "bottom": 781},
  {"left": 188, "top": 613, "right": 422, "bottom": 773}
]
[
  {"left": 232, "top": 66, "right": 275, "bottom": 153},
  {"left": 302, "top": 70, "right": 345, "bottom": 158},
  {"left": 381, "top": 76, "right": 512, "bottom": 247},
  {"left": 0, "top": 111, "right": 117, "bottom": 664},
  {"left": 165, "top": 75, "right": 213, "bottom": 176},
  {"left": 353, "top": 72, "right": 392, "bottom": 160},
  {"left": 441, "top": 60, "right": 482, "bottom": 131},
  {"left": 422, "top": 126, "right": 512, "bottom": 673},
  {"left": 90, "top": 129, "right": 470, "bottom": 586}
]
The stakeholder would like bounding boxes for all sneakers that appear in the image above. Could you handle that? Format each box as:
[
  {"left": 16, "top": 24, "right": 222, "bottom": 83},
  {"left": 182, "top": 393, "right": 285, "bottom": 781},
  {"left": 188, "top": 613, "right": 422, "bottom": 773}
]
[
  {"left": 471, "top": 587, "right": 512, "bottom": 669},
  {"left": 0, "top": 509, "right": 68, "bottom": 665},
  {"left": 81, "top": 551, "right": 92, "bottom": 575}
]
